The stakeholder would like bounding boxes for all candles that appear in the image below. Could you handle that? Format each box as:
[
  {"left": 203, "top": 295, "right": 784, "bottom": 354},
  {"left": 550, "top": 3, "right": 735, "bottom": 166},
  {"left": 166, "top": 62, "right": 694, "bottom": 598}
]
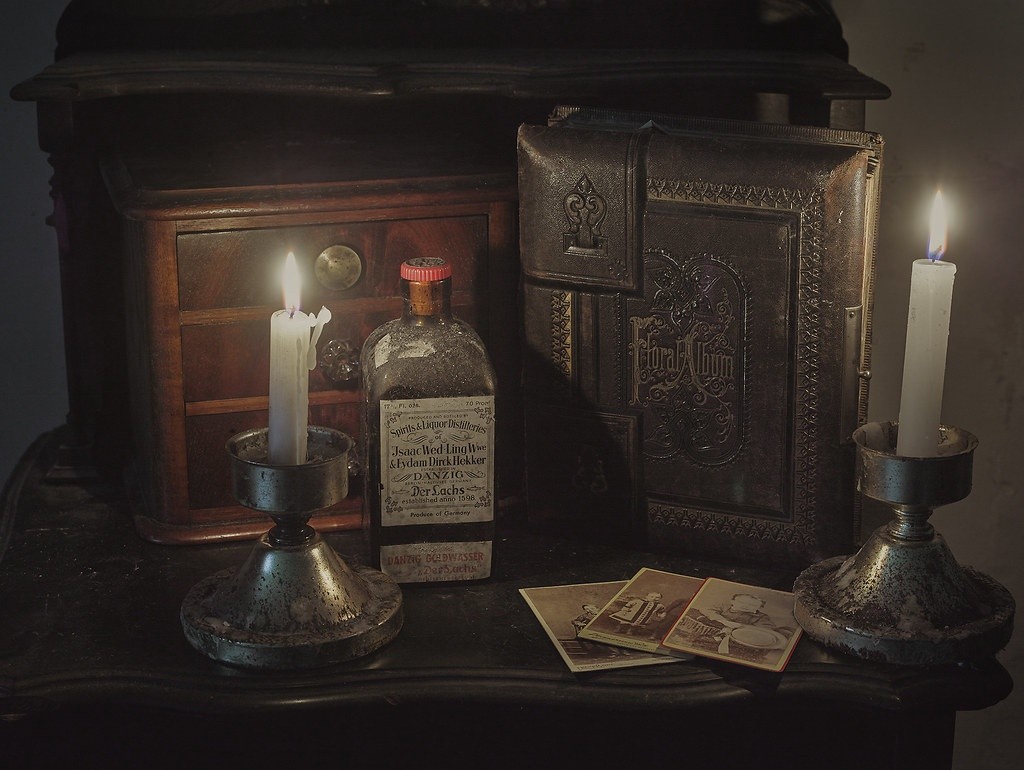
[
  {"left": 895, "top": 259, "right": 958, "bottom": 459},
  {"left": 269, "top": 307, "right": 335, "bottom": 464}
]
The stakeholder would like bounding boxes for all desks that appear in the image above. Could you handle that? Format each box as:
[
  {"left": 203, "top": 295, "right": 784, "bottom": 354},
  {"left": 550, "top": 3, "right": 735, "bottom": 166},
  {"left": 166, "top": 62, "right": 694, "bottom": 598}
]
[{"left": 0, "top": 497, "right": 1016, "bottom": 770}]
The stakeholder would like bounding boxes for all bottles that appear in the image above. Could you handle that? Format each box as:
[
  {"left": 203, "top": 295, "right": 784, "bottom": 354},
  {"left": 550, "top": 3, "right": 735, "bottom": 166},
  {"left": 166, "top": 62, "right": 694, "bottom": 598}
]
[{"left": 357, "top": 257, "right": 501, "bottom": 586}]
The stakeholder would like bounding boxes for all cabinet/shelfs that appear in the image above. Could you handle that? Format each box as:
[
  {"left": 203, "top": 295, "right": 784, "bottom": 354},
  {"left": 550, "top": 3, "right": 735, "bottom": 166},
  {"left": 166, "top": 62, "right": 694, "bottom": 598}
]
[{"left": 92, "top": 116, "right": 537, "bottom": 546}]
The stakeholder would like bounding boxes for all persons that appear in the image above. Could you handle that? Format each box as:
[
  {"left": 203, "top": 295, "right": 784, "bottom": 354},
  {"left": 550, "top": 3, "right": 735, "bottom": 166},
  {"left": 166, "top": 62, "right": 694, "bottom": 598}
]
[
  {"left": 571, "top": 605, "right": 631, "bottom": 658},
  {"left": 608, "top": 592, "right": 667, "bottom": 636},
  {"left": 674, "top": 593, "right": 776, "bottom": 662}
]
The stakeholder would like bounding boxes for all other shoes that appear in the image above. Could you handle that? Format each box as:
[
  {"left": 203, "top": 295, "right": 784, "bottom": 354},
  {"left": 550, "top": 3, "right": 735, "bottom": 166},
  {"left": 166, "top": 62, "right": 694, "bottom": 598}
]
[
  {"left": 627, "top": 632, "right": 632, "bottom": 635},
  {"left": 648, "top": 637, "right": 656, "bottom": 640},
  {"left": 614, "top": 629, "right": 620, "bottom": 632},
  {"left": 607, "top": 654, "right": 615, "bottom": 659}
]
